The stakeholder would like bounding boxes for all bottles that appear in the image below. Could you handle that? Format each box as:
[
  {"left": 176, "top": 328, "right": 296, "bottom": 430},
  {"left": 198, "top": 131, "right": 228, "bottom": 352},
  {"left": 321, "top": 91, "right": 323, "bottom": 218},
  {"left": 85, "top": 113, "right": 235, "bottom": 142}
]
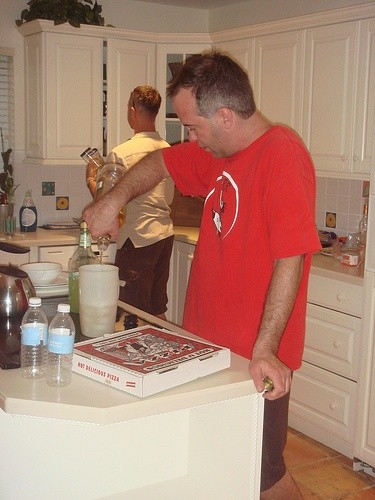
[
  {"left": 357, "top": 204, "right": 368, "bottom": 252},
  {"left": 68, "top": 221, "right": 101, "bottom": 314},
  {"left": 20, "top": 296, "right": 48, "bottom": 378},
  {"left": 47, "top": 303, "right": 76, "bottom": 387},
  {"left": 93, "top": 164, "right": 128, "bottom": 253},
  {"left": 19, "top": 189, "right": 37, "bottom": 232}
]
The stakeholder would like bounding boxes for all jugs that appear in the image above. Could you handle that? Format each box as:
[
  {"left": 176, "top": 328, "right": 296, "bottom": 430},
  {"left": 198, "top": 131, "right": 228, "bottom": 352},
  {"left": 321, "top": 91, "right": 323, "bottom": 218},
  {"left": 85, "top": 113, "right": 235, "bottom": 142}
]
[{"left": 0, "top": 264, "right": 37, "bottom": 369}]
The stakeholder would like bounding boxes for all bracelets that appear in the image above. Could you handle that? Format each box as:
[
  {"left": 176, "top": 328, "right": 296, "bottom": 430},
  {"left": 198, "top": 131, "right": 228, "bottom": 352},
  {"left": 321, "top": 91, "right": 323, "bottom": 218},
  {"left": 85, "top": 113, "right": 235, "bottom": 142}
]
[{"left": 87, "top": 179, "right": 96, "bottom": 187}]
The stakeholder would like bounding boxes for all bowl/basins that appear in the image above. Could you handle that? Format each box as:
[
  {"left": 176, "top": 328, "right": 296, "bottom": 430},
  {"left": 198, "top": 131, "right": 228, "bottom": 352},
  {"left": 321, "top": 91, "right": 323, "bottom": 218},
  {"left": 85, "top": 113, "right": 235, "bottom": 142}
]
[{"left": 20, "top": 262, "right": 63, "bottom": 286}]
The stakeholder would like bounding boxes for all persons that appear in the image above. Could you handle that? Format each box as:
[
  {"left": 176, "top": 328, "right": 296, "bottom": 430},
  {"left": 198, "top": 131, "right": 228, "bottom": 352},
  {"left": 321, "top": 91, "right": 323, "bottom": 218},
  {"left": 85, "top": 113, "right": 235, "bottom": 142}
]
[
  {"left": 86, "top": 85, "right": 176, "bottom": 322},
  {"left": 81, "top": 53, "right": 322, "bottom": 500}
]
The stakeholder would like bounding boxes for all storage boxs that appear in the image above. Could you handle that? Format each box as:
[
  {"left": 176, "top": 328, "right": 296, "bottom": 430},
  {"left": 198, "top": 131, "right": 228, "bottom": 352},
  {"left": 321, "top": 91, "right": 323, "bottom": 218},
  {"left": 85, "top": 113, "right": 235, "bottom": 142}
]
[{"left": 59, "top": 326, "right": 231, "bottom": 399}]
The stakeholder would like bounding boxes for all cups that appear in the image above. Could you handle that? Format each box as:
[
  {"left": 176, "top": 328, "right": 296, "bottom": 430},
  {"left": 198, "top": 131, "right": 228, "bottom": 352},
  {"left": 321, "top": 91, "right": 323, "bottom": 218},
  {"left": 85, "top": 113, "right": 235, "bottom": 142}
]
[
  {"left": 78, "top": 264, "right": 120, "bottom": 338},
  {"left": 346, "top": 232, "right": 362, "bottom": 250},
  {"left": 80, "top": 147, "right": 104, "bottom": 170}
]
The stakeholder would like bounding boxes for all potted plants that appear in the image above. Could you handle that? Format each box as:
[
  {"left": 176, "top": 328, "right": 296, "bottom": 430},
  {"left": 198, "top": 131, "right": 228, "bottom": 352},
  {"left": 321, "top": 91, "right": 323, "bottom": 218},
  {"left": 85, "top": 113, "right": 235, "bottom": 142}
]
[{"left": 0, "top": 128, "right": 21, "bottom": 231}]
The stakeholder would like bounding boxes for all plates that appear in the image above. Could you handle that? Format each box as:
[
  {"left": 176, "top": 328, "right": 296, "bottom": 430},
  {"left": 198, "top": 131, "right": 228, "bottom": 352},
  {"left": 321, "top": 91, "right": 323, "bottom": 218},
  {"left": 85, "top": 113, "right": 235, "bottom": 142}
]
[
  {"left": 320, "top": 247, "right": 337, "bottom": 256},
  {"left": 37, "top": 271, "right": 69, "bottom": 286}
]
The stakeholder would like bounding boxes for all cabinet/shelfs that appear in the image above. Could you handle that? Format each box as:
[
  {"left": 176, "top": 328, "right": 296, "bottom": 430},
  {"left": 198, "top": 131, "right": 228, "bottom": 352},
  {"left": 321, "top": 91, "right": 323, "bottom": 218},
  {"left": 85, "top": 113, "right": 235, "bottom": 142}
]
[
  {"left": 213, "top": 29, "right": 307, "bottom": 151},
  {"left": 157, "top": 42, "right": 213, "bottom": 144},
  {"left": 173, "top": 240, "right": 195, "bottom": 326},
  {"left": 37, "top": 243, "right": 77, "bottom": 271},
  {"left": 301, "top": 19, "right": 375, "bottom": 182},
  {"left": 287, "top": 273, "right": 361, "bottom": 450},
  {"left": 48, "top": 31, "right": 156, "bottom": 159}
]
